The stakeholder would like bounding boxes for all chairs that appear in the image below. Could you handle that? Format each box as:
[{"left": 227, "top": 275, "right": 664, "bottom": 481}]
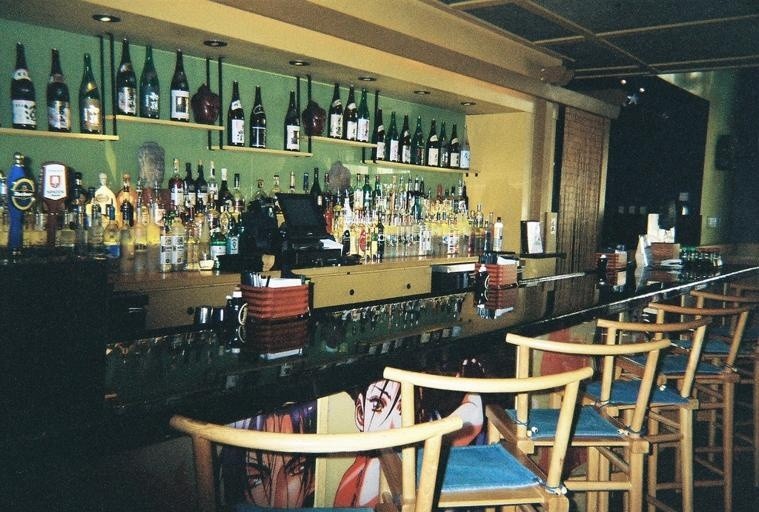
[
  {"left": 484, "top": 333, "right": 672, "bottom": 512},
  {"left": 547, "top": 316, "right": 713, "bottom": 512},
  {"left": 614, "top": 303, "right": 751, "bottom": 512},
  {"left": 378, "top": 366, "right": 594, "bottom": 512},
  {"left": 672, "top": 290, "right": 759, "bottom": 488},
  {"left": 708, "top": 281, "right": 759, "bottom": 340},
  {"left": 168, "top": 416, "right": 464, "bottom": 512}
]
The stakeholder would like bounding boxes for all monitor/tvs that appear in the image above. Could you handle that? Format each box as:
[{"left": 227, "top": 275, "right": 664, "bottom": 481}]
[{"left": 275, "top": 192, "right": 327, "bottom": 228}]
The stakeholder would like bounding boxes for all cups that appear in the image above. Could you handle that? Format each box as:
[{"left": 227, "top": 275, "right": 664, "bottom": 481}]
[{"left": 196, "top": 304, "right": 225, "bottom": 327}]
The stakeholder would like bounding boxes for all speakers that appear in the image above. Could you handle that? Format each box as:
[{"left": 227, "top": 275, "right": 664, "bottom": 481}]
[{"left": 718, "top": 135, "right": 737, "bottom": 170}]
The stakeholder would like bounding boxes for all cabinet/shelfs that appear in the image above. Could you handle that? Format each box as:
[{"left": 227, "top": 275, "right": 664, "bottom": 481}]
[{"left": 0, "top": 1, "right": 545, "bottom": 333}]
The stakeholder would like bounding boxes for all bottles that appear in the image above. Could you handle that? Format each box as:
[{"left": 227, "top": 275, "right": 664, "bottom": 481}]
[
  {"left": 249, "top": 180, "right": 267, "bottom": 202},
  {"left": 228, "top": 79, "right": 300, "bottom": 152},
  {"left": 597, "top": 254, "right": 608, "bottom": 273},
  {"left": 103, "top": 330, "right": 215, "bottom": 366},
  {"left": 327, "top": 82, "right": 370, "bottom": 142},
  {"left": 0, "top": 149, "right": 243, "bottom": 272},
  {"left": 596, "top": 273, "right": 608, "bottom": 287},
  {"left": 302, "top": 173, "right": 309, "bottom": 194},
  {"left": 269, "top": 175, "right": 282, "bottom": 199},
  {"left": 113, "top": 36, "right": 190, "bottom": 123},
  {"left": 226, "top": 286, "right": 248, "bottom": 326},
  {"left": 8, "top": 41, "right": 105, "bottom": 135},
  {"left": 288, "top": 171, "right": 297, "bottom": 194},
  {"left": 474, "top": 291, "right": 488, "bottom": 309},
  {"left": 474, "top": 263, "right": 489, "bottom": 290},
  {"left": 325, "top": 292, "right": 466, "bottom": 336},
  {"left": 301, "top": 99, "right": 327, "bottom": 136},
  {"left": 372, "top": 108, "right": 472, "bottom": 169},
  {"left": 311, "top": 168, "right": 503, "bottom": 253},
  {"left": 226, "top": 327, "right": 248, "bottom": 355},
  {"left": 191, "top": 84, "right": 222, "bottom": 125},
  {"left": 680, "top": 247, "right": 723, "bottom": 267}
]
